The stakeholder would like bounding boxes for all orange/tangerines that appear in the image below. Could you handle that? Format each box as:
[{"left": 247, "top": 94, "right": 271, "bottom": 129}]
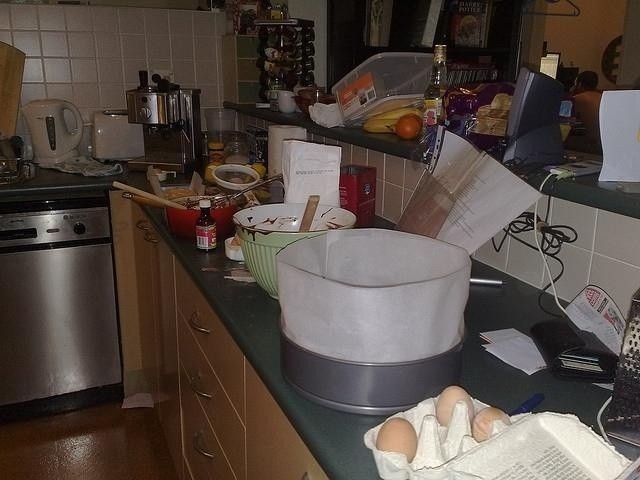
[
  {"left": 397, "top": 117, "right": 421, "bottom": 139},
  {"left": 400, "top": 114, "right": 423, "bottom": 130}
]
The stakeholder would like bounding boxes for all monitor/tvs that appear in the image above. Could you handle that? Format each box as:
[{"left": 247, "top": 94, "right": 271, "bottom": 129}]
[{"left": 546, "top": 52, "right": 561, "bottom": 67}]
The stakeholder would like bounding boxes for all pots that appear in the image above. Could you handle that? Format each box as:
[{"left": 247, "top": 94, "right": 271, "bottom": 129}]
[{"left": 121, "top": 192, "right": 238, "bottom": 240}]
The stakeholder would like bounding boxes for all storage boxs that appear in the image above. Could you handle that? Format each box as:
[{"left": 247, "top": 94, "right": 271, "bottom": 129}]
[{"left": 331, "top": 50, "right": 435, "bottom": 126}]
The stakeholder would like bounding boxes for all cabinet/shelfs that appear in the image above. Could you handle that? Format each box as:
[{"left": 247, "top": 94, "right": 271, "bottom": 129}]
[
  {"left": 221, "top": 34, "right": 268, "bottom": 105},
  {"left": 246, "top": 354, "right": 330, "bottom": 480},
  {"left": 130, "top": 202, "right": 183, "bottom": 476},
  {"left": 175, "top": 257, "right": 244, "bottom": 479}
]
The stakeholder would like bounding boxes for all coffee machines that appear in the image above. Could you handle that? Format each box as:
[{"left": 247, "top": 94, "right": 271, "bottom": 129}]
[{"left": 127, "top": 86, "right": 202, "bottom": 174}]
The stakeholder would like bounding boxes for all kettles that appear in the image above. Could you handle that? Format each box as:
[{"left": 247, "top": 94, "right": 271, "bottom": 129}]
[{"left": 21, "top": 99, "right": 84, "bottom": 164}]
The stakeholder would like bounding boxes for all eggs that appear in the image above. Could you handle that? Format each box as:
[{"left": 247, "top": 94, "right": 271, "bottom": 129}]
[
  {"left": 472, "top": 407, "right": 511, "bottom": 442},
  {"left": 437, "top": 385, "right": 473, "bottom": 426},
  {"left": 376, "top": 418, "right": 418, "bottom": 463}
]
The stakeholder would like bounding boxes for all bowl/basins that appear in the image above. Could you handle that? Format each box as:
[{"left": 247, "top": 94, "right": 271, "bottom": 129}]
[
  {"left": 225, "top": 237, "right": 245, "bottom": 262},
  {"left": 232, "top": 203, "right": 356, "bottom": 301}
]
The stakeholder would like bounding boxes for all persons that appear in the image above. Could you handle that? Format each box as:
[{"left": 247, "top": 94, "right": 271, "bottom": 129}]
[{"left": 568, "top": 69, "right": 606, "bottom": 155}]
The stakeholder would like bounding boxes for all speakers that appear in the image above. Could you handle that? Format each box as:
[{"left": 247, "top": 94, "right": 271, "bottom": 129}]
[{"left": 502, "top": 66, "right": 563, "bottom": 167}]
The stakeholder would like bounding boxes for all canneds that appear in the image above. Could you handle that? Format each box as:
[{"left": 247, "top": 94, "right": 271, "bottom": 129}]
[{"left": 208, "top": 142, "right": 225, "bottom": 164}]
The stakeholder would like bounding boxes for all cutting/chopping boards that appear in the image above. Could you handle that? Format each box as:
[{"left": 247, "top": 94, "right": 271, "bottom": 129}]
[{"left": 0, "top": 40, "right": 26, "bottom": 139}]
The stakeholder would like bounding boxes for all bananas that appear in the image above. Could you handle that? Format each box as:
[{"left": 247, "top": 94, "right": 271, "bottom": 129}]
[{"left": 364, "top": 107, "right": 422, "bottom": 133}]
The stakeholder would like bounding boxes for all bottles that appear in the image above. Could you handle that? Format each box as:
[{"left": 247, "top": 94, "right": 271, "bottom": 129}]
[
  {"left": 423, "top": 45, "right": 449, "bottom": 127},
  {"left": 195, "top": 199, "right": 217, "bottom": 253},
  {"left": 223, "top": 130, "right": 251, "bottom": 165}
]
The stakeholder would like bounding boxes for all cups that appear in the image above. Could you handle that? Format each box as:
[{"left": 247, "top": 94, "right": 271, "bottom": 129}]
[
  {"left": 204, "top": 107, "right": 236, "bottom": 164},
  {"left": 278, "top": 91, "right": 297, "bottom": 114}
]
